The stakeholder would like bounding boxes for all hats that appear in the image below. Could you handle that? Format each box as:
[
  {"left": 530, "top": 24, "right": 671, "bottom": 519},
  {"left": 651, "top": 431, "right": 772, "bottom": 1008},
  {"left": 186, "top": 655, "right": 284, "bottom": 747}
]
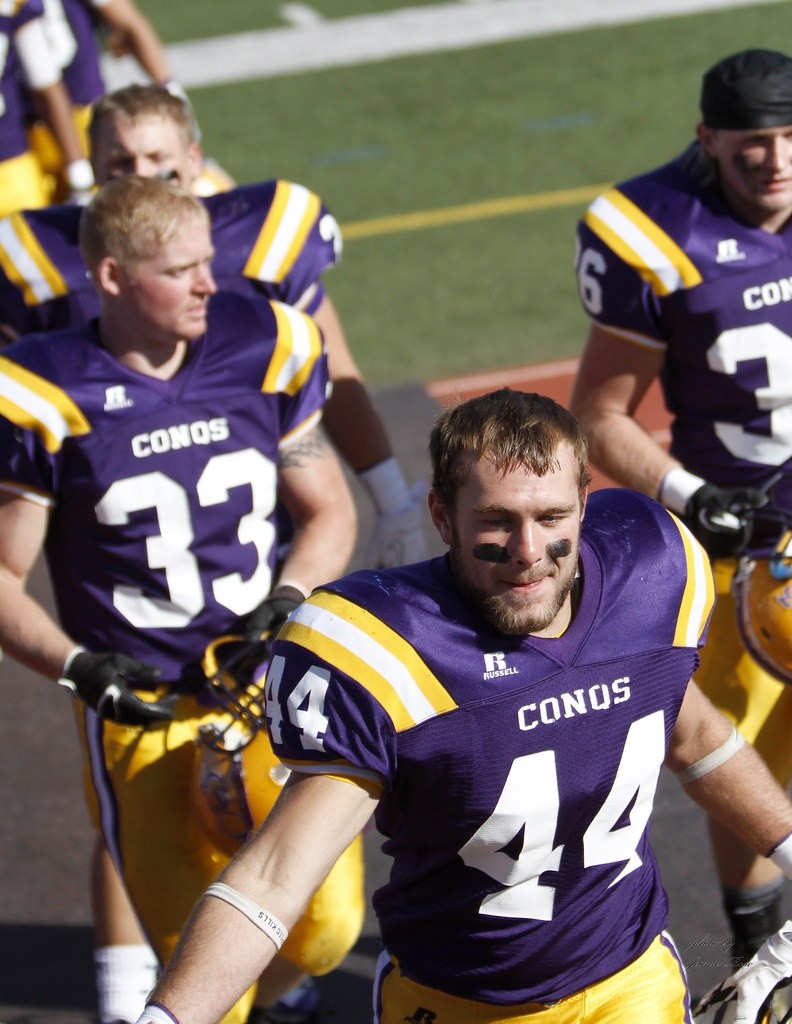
[{"left": 700, "top": 48, "right": 791, "bottom": 130}]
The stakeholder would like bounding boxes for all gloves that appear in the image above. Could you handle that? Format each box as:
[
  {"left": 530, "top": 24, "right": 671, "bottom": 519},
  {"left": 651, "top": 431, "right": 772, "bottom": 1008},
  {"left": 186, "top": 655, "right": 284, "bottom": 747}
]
[
  {"left": 185, "top": 583, "right": 307, "bottom": 678},
  {"left": 60, "top": 646, "right": 179, "bottom": 725},
  {"left": 659, "top": 466, "right": 769, "bottom": 554}
]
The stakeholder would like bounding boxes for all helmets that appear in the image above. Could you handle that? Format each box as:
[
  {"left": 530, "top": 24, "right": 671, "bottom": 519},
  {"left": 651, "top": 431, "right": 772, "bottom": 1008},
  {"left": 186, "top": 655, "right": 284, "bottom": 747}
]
[
  {"left": 181, "top": 636, "right": 292, "bottom": 858},
  {"left": 732, "top": 455, "right": 791, "bottom": 681}
]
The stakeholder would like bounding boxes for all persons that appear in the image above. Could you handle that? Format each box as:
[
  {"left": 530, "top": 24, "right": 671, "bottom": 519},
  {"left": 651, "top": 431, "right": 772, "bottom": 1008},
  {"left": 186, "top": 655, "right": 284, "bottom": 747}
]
[
  {"left": 0, "top": 174, "right": 369, "bottom": 1022},
  {"left": 137, "top": 387, "right": 792, "bottom": 1024},
  {"left": 2, "top": 84, "right": 431, "bottom": 1022},
  {"left": 566, "top": 48, "right": 792, "bottom": 966},
  {"left": 0, "top": 0, "right": 237, "bottom": 211}
]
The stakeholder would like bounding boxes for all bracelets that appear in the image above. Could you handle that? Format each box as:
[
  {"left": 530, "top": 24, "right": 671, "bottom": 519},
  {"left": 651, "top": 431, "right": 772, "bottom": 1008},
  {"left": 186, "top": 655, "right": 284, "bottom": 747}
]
[{"left": 202, "top": 881, "right": 289, "bottom": 953}]
